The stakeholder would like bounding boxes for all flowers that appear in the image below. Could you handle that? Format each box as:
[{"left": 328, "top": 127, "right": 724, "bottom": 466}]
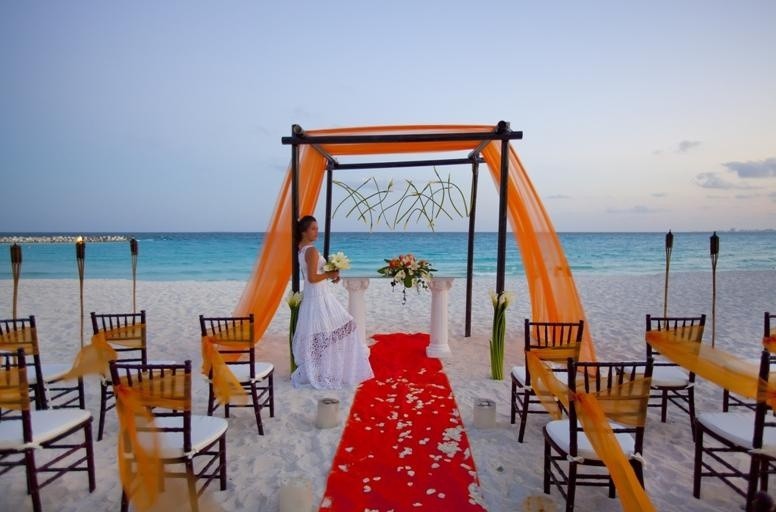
[
  {"left": 376, "top": 251, "right": 437, "bottom": 305},
  {"left": 322, "top": 250, "right": 353, "bottom": 284},
  {"left": 487, "top": 288, "right": 512, "bottom": 380},
  {"left": 284, "top": 289, "right": 304, "bottom": 374}
]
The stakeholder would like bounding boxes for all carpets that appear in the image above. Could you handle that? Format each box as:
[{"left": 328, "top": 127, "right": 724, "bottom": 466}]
[{"left": 317, "top": 332, "right": 488, "bottom": 512}]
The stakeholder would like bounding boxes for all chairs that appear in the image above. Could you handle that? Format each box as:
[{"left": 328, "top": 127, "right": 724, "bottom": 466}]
[
  {"left": 544, "top": 358, "right": 654, "bottom": 512},
  {"left": 200, "top": 315, "right": 274, "bottom": 436},
  {"left": 110, "top": 360, "right": 230, "bottom": 512},
  {"left": 511, "top": 318, "right": 585, "bottom": 442},
  {"left": 694, "top": 349, "right": 776, "bottom": 511},
  {"left": 723, "top": 312, "right": 776, "bottom": 414},
  {"left": 1, "top": 309, "right": 85, "bottom": 411},
  {"left": 91, "top": 312, "right": 179, "bottom": 440},
  {"left": 1, "top": 348, "right": 95, "bottom": 512},
  {"left": 614, "top": 314, "right": 706, "bottom": 423}
]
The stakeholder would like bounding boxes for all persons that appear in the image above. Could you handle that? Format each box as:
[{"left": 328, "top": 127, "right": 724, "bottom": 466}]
[{"left": 292, "top": 216, "right": 375, "bottom": 391}]
